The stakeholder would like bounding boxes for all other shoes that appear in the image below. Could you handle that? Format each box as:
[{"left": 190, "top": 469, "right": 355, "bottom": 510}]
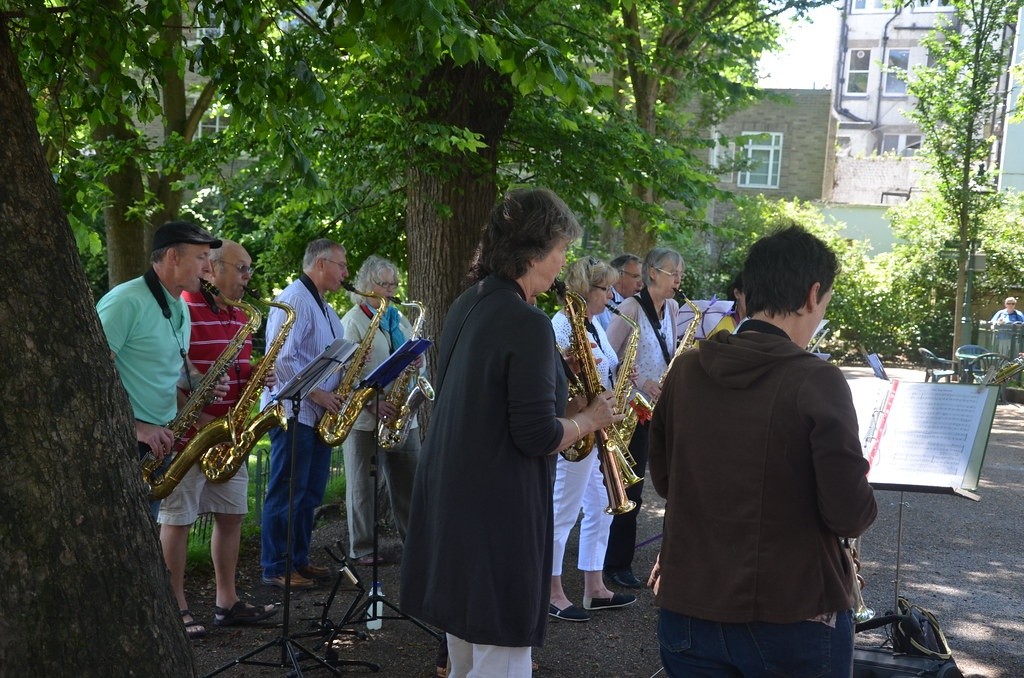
[
  {"left": 608, "top": 566, "right": 641, "bottom": 588},
  {"left": 356, "top": 553, "right": 385, "bottom": 566},
  {"left": 532, "top": 662, "right": 538, "bottom": 671},
  {"left": 549, "top": 603, "right": 591, "bottom": 621},
  {"left": 583, "top": 591, "right": 637, "bottom": 610},
  {"left": 437, "top": 666, "right": 448, "bottom": 678}
]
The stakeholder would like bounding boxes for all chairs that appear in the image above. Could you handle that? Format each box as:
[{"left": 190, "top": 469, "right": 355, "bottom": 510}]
[
  {"left": 918, "top": 347, "right": 961, "bottom": 383},
  {"left": 955, "top": 344, "right": 989, "bottom": 383},
  {"left": 968, "top": 352, "right": 1011, "bottom": 402}
]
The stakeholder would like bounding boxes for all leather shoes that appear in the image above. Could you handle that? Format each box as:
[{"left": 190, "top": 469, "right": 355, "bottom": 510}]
[
  {"left": 260, "top": 569, "right": 314, "bottom": 589},
  {"left": 296, "top": 562, "right": 332, "bottom": 578}
]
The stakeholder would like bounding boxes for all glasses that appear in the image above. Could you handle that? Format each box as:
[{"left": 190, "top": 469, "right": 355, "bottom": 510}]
[
  {"left": 591, "top": 285, "right": 612, "bottom": 291},
  {"left": 587, "top": 256, "right": 599, "bottom": 269},
  {"left": 373, "top": 279, "right": 398, "bottom": 289},
  {"left": 623, "top": 271, "right": 641, "bottom": 281},
  {"left": 324, "top": 259, "right": 351, "bottom": 272},
  {"left": 1006, "top": 303, "right": 1015, "bottom": 305},
  {"left": 655, "top": 267, "right": 683, "bottom": 277},
  {"left": 220, "top": 261, "right": 252, "bottom": 274}
]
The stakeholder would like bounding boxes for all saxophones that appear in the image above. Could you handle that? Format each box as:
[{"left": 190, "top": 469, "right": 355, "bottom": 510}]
[
  {"left": 376, "top": 296, "right": 436, "bottom": 452},
  {"left": 315, "top": 280, "right": 388, "bottom": 447},
  {"left": 646, "top": 288, "right": 704, "bottom": 422},
  {"left": 198, "top": 285, "right": 297, "bottom": 484},
  {"left": 610, "top": 305, "right": 651, "bottom": 455},
  {"left": 840, "top": 536, "right": 876, "bottom": 625},
  {"left": 548, "top": 277, "right": 645, "bottom": 516},
  {"left": 139, "top": 276, "right": 262, "bottom": 499}
]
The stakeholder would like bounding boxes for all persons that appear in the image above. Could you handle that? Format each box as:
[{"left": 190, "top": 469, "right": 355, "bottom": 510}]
[
  {"left": 398, "top": 188, "right": 628, "bottom": 678},
  {"left": 95, "top": 222, "right": 223, "bottom": 526},
  {"left": 648, "top": 228, "right": 880, "bottom": 678},
  {"left": 258, "top": 239, "right": 354, "bottom": 589},
  {"left": 990, "top": 297, "right": 1024, "bottom": 361},
  {"left": 156, "top": 237, "right": 280, "bottom": 637},
  {"left": 340, "top": 253, "right": 428, "bottom": 567},
  {"left": 437, "top": 246, "right": 747, "bottom": 678}
]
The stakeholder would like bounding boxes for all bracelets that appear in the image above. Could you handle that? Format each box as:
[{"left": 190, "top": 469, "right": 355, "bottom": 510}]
[{"left": 568, "top": 418, "right": 580, "bottom": 443}]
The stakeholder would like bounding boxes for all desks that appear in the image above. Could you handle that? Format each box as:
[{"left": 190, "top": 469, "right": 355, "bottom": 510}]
[{"left": 958, "top": 353, "right": 1001, "bottom": 374}]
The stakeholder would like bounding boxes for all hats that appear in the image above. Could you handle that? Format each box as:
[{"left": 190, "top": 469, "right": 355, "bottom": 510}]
[{"left": 152, "top": 221, "right": 222, "bottom": 252}]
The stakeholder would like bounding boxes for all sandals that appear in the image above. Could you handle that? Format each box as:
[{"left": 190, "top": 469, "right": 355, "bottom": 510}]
[
  {"left": 213, "top": 599, "right": 280, "bottom": 626},
  {"left": 179, "top": 609, "right": 207, "bottom": 638}
]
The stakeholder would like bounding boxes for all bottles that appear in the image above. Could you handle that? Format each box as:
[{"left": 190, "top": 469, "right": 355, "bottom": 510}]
[{"left": 366, "top": 581, "right": 383, "bottom": 630}]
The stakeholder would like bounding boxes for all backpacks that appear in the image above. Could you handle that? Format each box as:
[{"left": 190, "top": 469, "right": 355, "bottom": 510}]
[{"left": 885, "top": 596, "right": 955, "bottom": 663}]
